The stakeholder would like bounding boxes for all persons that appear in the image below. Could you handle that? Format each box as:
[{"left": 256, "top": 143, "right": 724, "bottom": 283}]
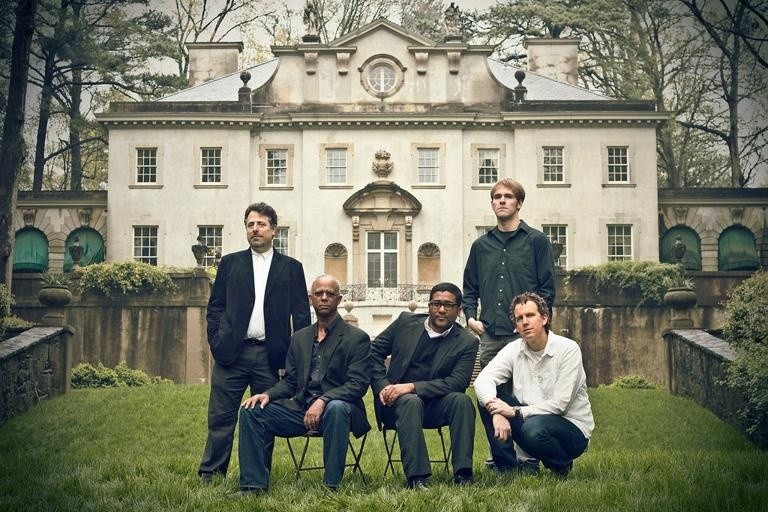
[
  {"left": 462, "top": 176, "right": 556, "bottom": 480},
  {"left": 472, "top": 289, "right": 596, "bottom": 481},
  {"left": 196, "top": 202, "right": 311, "bottom": 484},
  {"left": 370, "top": 282, "right": 479, "bottom": 495},
  {"left": 225, "top": 273, "right": 370, "bottom": 497}
]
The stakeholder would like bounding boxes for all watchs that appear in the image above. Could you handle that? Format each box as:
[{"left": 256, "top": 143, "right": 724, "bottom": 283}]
[{"left": 513, "top": 405, "right": 521, "bottom": 418}]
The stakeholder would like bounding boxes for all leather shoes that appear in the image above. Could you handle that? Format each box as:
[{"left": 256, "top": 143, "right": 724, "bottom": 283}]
[{"left": 518, "top": 458, "right": 540, "bottom": 476}]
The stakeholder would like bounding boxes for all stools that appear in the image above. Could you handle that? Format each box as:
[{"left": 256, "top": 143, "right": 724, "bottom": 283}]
[
  {"left": 280, "top": 427, "right": 372, "bottom": 485},
  {"left": 379, "top": 422, "right": 455, "bottom": 480}
]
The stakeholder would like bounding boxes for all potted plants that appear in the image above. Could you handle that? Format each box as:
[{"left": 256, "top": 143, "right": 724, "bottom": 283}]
[
  {"left": 35, "top": 269, "right": 74, "bottom": 323},
  {"left": 662, "top": 266, "right": 701, "bottom": 331}
]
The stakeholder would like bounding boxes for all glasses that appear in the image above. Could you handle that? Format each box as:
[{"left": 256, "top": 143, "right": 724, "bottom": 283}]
[{"left": 430, "top": 301, "right": 457, "bottom": 311}]
